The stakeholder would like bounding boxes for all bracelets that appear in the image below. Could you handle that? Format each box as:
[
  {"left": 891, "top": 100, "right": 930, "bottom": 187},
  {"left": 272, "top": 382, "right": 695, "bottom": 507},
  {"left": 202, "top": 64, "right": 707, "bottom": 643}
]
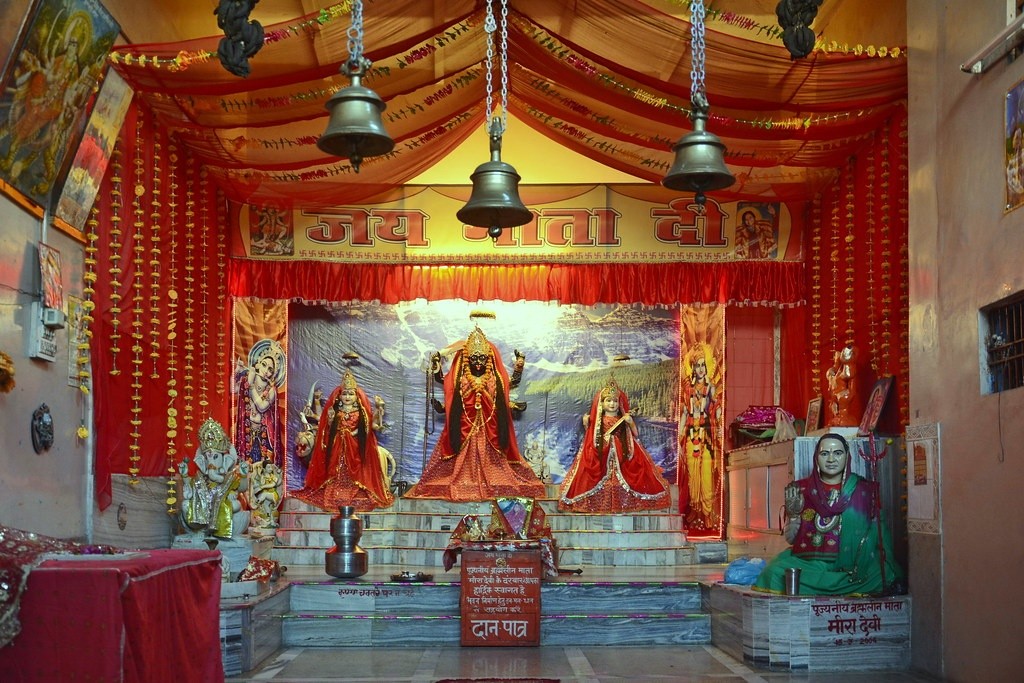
[
  {"left": 240, "top": 474, "right": 248, "bottom": 478},
  {"left": 514, "top": 365, "right": 523, "bottom": 373},
  {"left": 508, "top": 401, "right": 515, "bottom": 409},
  {"left": 181, "top": 474, "right": 189, "bottom": 478},
  {"left": 629, "top": 422, "right": 635, "bottom": 428}
]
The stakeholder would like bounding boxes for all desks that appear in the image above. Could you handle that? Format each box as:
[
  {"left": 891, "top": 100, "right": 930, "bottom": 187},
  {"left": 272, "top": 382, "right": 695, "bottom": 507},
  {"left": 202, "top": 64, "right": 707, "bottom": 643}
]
[{"left": 2, "top": 543, "right": 227, "bottom": 683}]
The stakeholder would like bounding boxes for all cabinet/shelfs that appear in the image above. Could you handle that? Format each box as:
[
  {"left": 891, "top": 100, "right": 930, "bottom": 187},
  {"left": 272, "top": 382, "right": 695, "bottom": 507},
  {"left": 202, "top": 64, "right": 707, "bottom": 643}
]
[{"left": 728, "top": 433, "right": 891, "bottom": 536}]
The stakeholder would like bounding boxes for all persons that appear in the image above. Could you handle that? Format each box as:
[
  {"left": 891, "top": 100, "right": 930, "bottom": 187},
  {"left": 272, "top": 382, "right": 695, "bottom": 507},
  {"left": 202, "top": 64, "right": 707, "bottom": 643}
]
[
  {"left": 294, "top": 371, "right": 394, "bottom": 507},
  {"left": 253, "top": 456, "right": 279, "bottom": 516},
  {"left": 562, "top": 382, "right": 672, "bottom": 512},
  {"left": 763, "top": 434, "right": 904, "bottom": 597},
  {"left": 177, "top": 439, "right": 250, "bottom": 528},
  {"left": 429, "top": 339, "right": 528, "bottom": 486}
]
